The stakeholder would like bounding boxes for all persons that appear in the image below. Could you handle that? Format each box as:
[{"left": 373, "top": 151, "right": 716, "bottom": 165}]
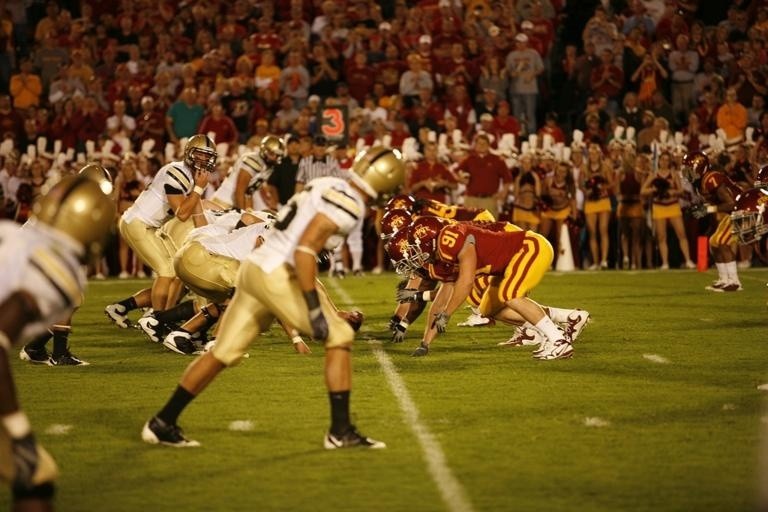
[
  {"left": 615, "top": 150, "right": 644, "bottom": 272},
  {"left": 385, "top": 226, "right": 591, "bottom": 345},
  {"left": 580, "top": 144, "right": 616, "bottom": 270},
  {"left": 114, "top": 160, "right": 148, "bottom": 279},
  {"left": 296, "top": 137, "right": 342, "bottom": 191},
  {"left": 405, "top": 143, "right": 457, "bottom": 204},
  {"left": 643, "top": 152, "right": 696, "bottom": 268},
  {"left": 380, "top": 210, "right": 542, "bottom": 346},
  {"left": 541, "top": 161, "right": 578, "bottom": 269},
  {"left": 406, "top": 217, "right": 574, "bottom": 362},
  {"left": 385, "top": 195, "right": 497, "bottom": 328},
  {"left": 209, "top": 134, "right": 286, "bottom": 214},
  {"left": 270, "top": 138, "right": 302, "bottom": 204},
  {"left": 757, "top": 165, "right": 767, "bottom": 183},
  {"left": 138, "top": 208, "right": 315, "bottom": 353},
  {"left": 2, "top": 0, "right": 554, "bottom": 140},
  {"left": 155, "top": 201, "right": 226, "bottom": 339},
  {"left": 0, "top": 158, "right": 48, "bottom": 224},
  {"left": 140, "top": 145, "right": 406, "bottom": 452},
  {"left": 683, "top": 152, "right": 745, "bottom": 292},
  {"left": 105, "top": 135, "right": 217, "bottom": 328},
  {"left": 447, "top": 135, "right": 513, "bottom": 218},
  {"left": 19, "top": 165, "right": 112, "bottom": 369},
  {"left": 728, "top": 188, "right": 768, "bottom": 391},
  {"left": 534, "top": 0, "right": 768, "bottom": 147},
  {"left": 138, "top": 156, "right": 161, "bottom": 279},
  {"left": 0, "top": 174, "right": 120, "bottom": 511},
  {"left": 163, "top": 219, "right": 360, "bottom": 360},
  {"left": 511, "top": 155, "right": 541, "bottom": 232}
]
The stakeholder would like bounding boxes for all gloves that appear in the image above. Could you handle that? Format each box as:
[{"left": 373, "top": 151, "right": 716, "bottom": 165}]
[
  {"left": 389, "top": 317, "right": 400, "bottom": 331},
  {"left": 391, "top": 326, "right": 406, "bottom": 343},
  {"left": 309, "top": 308, "right": 328, "bottom": 341},
  {"left": 397, "top": 290, "right": 423, "bottom": 304},
  {"left": 8, "top": 434, "right": 58, "bottom": 497},
  {"left": 430, "top": 311, "right": 449, "bottom": 333},
  {"left": 409, "top": 344, "right": 428, "bottom": 356}
]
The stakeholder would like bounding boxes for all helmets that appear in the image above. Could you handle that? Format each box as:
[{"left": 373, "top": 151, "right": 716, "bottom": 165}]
[
  {"left": 259, "top": 136, "right": 285, "bottom": 163},
  {"left": 184, "top": 136, "right": 217, "bottom": 173},
  {"left": 682, "top": 151, "right": 709, "bottom": 181},
  {"left": 729, "top": 187, "right": 768, "bottom": 246},
  {"left": 409, "top": 217, "right": 443, "bottom": 268},
  {"left": 349, "top": 147, "right": 404, "bottom": 196},
  {"left": 381, "top": 211, "right": 412, "bottom": 241},
  {"left": 388, "top": 196, "right": 416, "bottom": 217},
  {"left": 78, "top": 166, "right": 113, "bottom": 196},
  {"left": 385, "top": 228, "right": 418, "bottom": 276},
  {"left": 37, "top": 175, "right": 112, "bottom": 253}
]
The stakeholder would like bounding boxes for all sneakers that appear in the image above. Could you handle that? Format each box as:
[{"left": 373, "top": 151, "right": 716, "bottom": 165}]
[
  {"left": 138, "top": 315, "right": 162, "bottom": 343},
  {"left": 563, "top": 309, "right": 591, "bottom": 344},
  {"left": 714, "top": 283, "right": 741, "bottom": 293},
  {"left": 48, "top": 357, "right": 89, "bottom": 367},
  {"left": 705, "top": 281, "right": 728, "bottom": 290},
  {"left": 533, "top": 338, "right": 574, "bottom": 360},
  {"left": 142, "top": 418, "right": 199, "bottom": 448},
  {"left": 497, "top": 329, "right": 544, "bottom": 347},
  {"left": 457, "top": 313, "right": 491, "bottom": 327},
  {"left": 104, "top": 304, "right": 131, "bottom": 328},
  {"left": 163, "top": 330, "right": 200, "bottom": 355},
  {"left": 19, "top": 348, "right": 53, "bottom": 365},
  {"left": 324, "top": 430, "right": 386, "bottom": 450}
]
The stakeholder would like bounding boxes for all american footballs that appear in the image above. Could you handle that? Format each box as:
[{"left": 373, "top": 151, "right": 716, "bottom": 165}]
[{"left": 348, "top": 311, "right": 363, "bottom": 332}]
